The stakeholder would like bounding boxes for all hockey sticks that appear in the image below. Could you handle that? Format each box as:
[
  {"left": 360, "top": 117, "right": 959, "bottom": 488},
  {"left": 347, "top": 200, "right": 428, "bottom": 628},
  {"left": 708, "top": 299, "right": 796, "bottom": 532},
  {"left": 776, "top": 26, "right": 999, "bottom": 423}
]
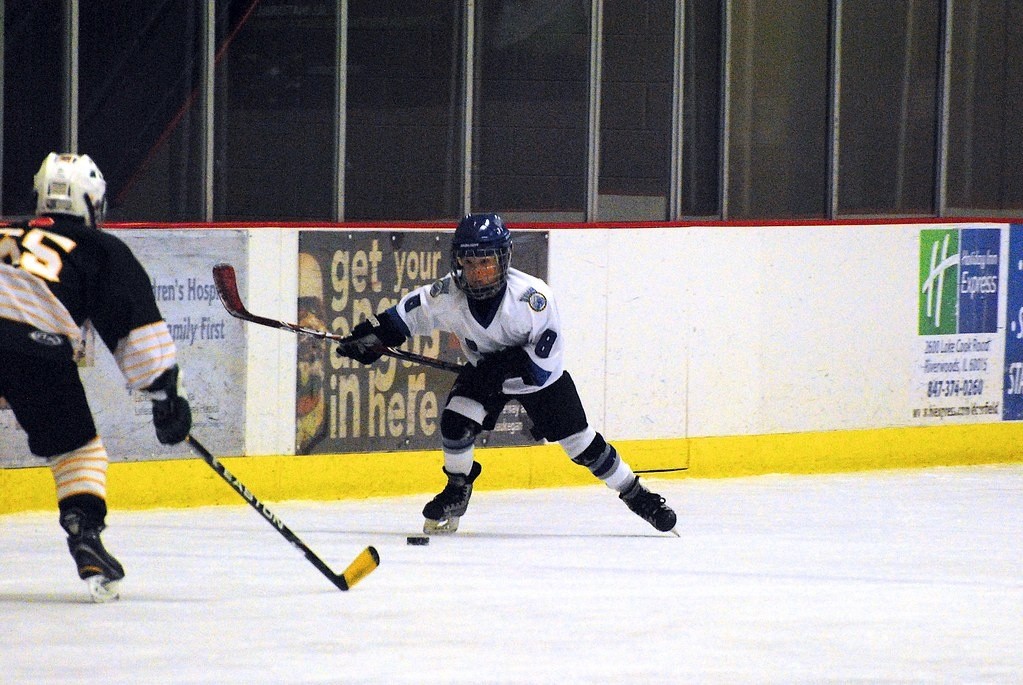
[
  {"left": 182, "top": 433, "right": 381, "bottom": 591},
  {"left": 212, "top": 262, "right": 480, "bottom": 376}
]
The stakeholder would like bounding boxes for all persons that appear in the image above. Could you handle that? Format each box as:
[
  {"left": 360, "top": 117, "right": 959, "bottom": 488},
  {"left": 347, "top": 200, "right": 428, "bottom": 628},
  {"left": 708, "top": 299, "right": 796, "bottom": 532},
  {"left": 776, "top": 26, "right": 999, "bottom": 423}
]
[
  {"left": 0, "top": 150, "right": 192, "bottom": 603},
  {"left": 335, "top": 213, "right": 681, "bottom": 537}
]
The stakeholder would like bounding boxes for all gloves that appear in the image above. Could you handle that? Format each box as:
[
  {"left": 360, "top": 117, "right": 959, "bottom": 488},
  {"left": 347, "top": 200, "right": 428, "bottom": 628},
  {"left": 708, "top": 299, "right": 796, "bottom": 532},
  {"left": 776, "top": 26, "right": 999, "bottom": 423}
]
[
  {"left": 477, "top": 349, "right": 517, "bottom": 394},
  {"left": 338, "top": 313, "right": 405, "bottom": 365},
  {"left": 153, "top": 388, "right": 191, "bottom": 445}
]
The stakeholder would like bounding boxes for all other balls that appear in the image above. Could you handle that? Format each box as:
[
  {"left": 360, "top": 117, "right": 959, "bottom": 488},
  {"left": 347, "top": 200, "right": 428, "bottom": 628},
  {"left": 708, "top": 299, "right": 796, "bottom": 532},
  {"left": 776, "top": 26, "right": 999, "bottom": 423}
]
[{"left": 406, "top": 536, "right": 431, "bottom": 548}]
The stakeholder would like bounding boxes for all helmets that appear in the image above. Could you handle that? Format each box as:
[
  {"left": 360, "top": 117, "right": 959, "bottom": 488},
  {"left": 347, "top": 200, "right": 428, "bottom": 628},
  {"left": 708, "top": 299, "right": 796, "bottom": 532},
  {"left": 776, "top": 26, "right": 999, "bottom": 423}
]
[
  {"left": 450, "top": 213, "right": 513, "bottom": 300},
  {"left": 34, "top": 151, "right": 109, "bottom": 229}
]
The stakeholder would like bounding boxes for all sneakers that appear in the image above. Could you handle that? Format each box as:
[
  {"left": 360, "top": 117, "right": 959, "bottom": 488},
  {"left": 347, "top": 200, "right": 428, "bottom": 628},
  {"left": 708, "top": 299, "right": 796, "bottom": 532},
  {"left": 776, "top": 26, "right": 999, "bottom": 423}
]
[
  {"left": 620, "top": 475, "right": 683, "bottom": 539},
  {"left": 62, "top": 506, "right": 126, "bottom": 604},
  {"left": 422, "top": 461, "right": 481, "bottom": 536}
]
